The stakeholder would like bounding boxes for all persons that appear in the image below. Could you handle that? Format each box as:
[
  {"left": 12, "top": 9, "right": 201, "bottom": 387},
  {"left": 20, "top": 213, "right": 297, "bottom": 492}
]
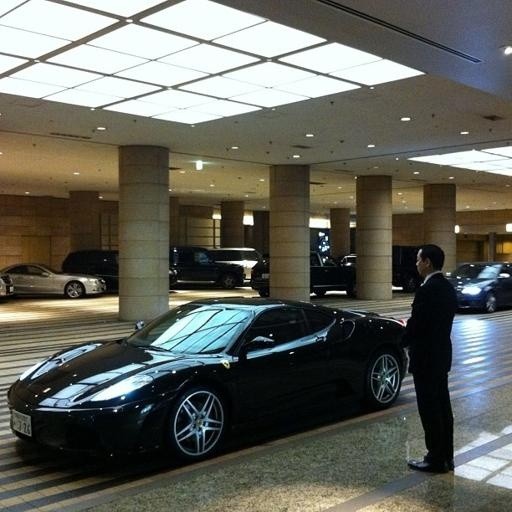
[{"left": 392, "top": 243, "right": 456, "bottom": 473}]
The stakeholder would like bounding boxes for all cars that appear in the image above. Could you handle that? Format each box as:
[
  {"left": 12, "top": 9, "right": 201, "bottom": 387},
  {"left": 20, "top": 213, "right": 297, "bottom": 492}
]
[
  {"left": 448, "top": 261, "right": 511, "bottom": 314},
  {"left": 0, "top": 262, "right": 107, "bottom": 299},
  {"left": 176, "top": 244, "right": 356, "bottom": 297},
  {"left": 8, "top": 298, "right": 407, "bottom": 462}
]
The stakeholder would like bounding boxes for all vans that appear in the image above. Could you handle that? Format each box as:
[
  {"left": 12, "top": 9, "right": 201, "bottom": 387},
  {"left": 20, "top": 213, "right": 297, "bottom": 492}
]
[{"left": 63, "top": 249, "right": 178, "bottom": 288}]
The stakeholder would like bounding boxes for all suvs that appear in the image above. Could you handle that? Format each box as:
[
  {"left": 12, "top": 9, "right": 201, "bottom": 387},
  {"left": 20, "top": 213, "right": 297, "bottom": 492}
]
[{"left": 342, "top": 245, "right": 422, "bottom": 290}]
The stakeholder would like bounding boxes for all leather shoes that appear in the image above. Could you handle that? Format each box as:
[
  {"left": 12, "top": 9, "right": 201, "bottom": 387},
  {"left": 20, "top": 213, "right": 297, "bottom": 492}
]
[{"left": 408, "top": 460, "right": 455, "bottom": 472}]
